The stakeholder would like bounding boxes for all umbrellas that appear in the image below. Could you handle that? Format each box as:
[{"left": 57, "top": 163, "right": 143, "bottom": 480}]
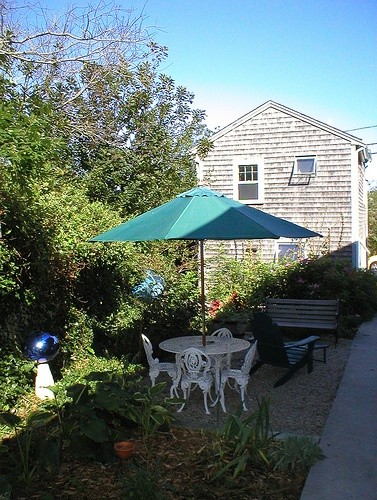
[{"left": 88, "top": 185, "right": 324, "bottom": 347}]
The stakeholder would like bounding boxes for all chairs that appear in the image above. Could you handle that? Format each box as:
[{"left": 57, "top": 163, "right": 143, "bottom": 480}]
[
  {"left": 250, "top": 312, "right": 320, "bottom": 388},
  {"left": 141, "top": 328, "right": 257, "bottom": 414}
]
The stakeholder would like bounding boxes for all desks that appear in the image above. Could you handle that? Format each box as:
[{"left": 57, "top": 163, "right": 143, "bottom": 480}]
[{"left": 159, "top": 336, "right": 250, "bottom": 413}]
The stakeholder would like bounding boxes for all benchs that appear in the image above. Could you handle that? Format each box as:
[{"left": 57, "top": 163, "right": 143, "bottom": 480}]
[{"left": 264, "top": 298, "right": 341, "bottom": 350}]
